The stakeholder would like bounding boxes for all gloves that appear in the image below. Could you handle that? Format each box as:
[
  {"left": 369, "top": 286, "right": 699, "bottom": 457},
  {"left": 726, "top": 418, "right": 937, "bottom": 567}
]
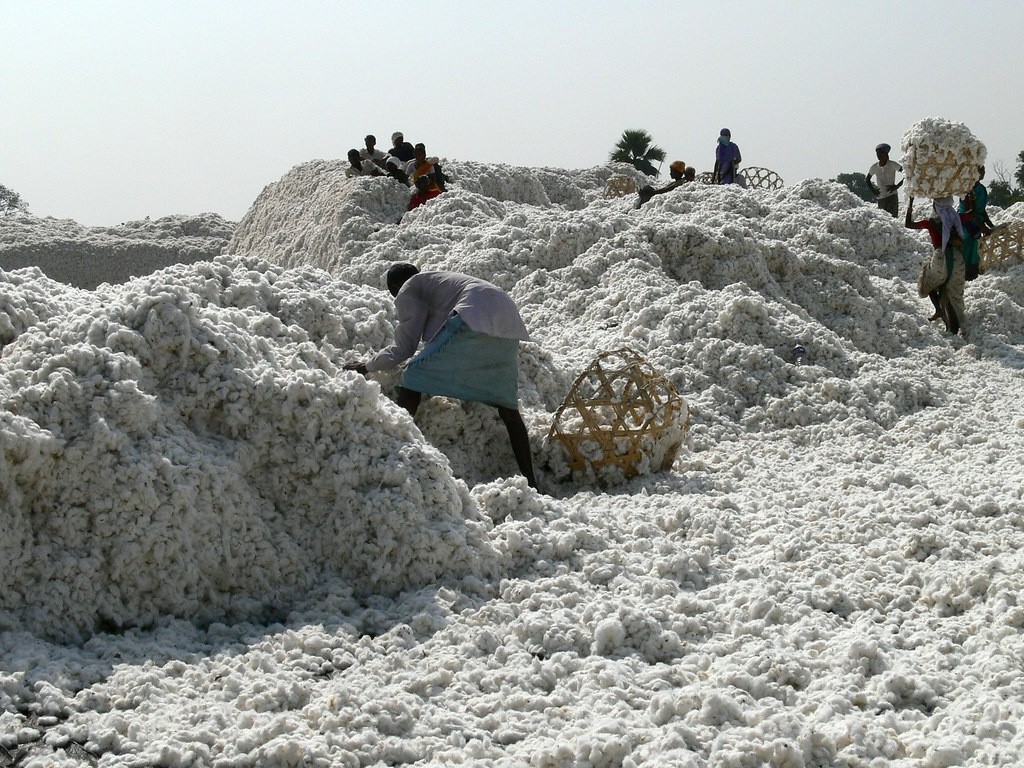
[{"left": 342, "top": 361, "right": 369, "bottom": 376}]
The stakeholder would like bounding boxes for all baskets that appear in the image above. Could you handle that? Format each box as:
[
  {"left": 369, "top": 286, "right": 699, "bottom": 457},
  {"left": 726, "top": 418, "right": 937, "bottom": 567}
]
[
  {"left": 905, "top": 143, "right": 975, "bottom": 195},
  {"left": 977, "top": 221, "right": 1024, "bottom": 272},
  {"left": 548, "top": 348, "right": 689, "bottom": 489},
  {"left": 602, "top": 178, "right": 636, "bottom": 200},
  {"left": 694, "top": 167, "right": 783, "bottom": 190}
]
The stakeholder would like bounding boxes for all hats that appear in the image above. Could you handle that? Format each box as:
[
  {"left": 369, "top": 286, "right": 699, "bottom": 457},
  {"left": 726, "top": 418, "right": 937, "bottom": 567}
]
[
  {"left": 671, "top": 161, "right": 685, "bottom": 174},
  {"left": 392, "top": 132, "right": 403, "bottom": 142},
  {"left": 684, "top": 167, "right": 695, "bottom": 177},
  {"left": 386, "top": 156, "right": 401, "bottom": 169},
  {"left": 720, "top": 129, "right": 730, "bottom": 136},
  {"left": 387, "top": 264, "right": 419, "bottom": 298},
  {"left": 876, "top": 144, "right": 891, "bottom": 153}
]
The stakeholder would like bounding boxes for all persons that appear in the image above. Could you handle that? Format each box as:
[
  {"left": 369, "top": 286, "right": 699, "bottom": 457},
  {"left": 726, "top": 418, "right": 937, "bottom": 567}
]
[
  {"left": 344, "top": 129, "right": 446, "bottom": 212},
  {"left": 866, "top": 143, "right": 904, "bottom": 218},
  {"left": 710, "top": 128, "right": 742, "bottom": 186},
  {"left": 637, "top": 128, "right": 697, "bottom": 206},
  {"left": 905, "top": 165, "right": 996, "bottom": 334},
  {"left": 344, "top": 264, "right": 533, "bottom": 493}
]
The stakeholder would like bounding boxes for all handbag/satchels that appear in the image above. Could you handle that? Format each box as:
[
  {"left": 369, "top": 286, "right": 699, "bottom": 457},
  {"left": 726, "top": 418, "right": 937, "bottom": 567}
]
[{"left": 917, "top": 248, "right": 948, "bottom": 298}]
